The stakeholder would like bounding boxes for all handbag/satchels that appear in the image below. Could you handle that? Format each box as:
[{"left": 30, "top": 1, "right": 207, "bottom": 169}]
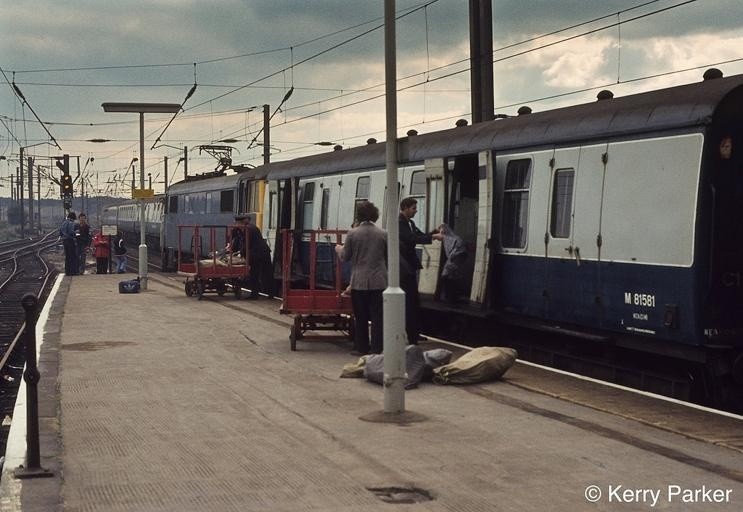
[{"left": 119, "top": 276, "right": 140, "bottom": 293}]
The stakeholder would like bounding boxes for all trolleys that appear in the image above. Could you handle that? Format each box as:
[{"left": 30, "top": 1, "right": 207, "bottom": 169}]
[
  {"left": 177, "top": 223, "right": 250, "bottom": 301},
  {"left": 279, "top": 229, "right": 356, "bottom": 352}
]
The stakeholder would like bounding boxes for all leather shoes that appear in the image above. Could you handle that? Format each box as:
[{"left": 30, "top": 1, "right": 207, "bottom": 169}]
[{"left": 417, "top": 335, "right": 427, "bottom": 340}]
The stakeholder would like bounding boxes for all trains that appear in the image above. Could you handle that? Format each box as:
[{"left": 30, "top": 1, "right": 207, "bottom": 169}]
[
  {"left": 100, "top": 193, "right": 165, "bottom": 251},
  {"left": 158, "top": 67, "right": 743, "bottom": 384}
]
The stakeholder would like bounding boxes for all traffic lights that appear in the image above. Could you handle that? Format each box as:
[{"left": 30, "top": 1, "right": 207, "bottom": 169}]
[{"left": 62, "top": 175, "right": 72, "bottom": 194}]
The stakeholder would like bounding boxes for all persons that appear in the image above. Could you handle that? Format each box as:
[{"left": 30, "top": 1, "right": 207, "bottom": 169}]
[
  {"left": 334, "top": 197, "right": 444, "bottom": 356},
  {"left": 114, "top": 230, "right": 128, "bottom": 274},
  {"left": 76, "top": 213, "right": 89, "bottom": 275},
  {"left": 58, "top": 211, "right": 79, "bottom": 275},
  {"left": 94, "top": 226, "right": 110, "bottom": 273},
  {"left": 227, "top": 214, "right": 275, "bottom": 299}
]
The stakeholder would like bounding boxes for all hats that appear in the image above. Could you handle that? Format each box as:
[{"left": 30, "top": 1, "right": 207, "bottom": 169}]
[{"left": 236, "top": 215, "right": 249, "bottom": 220}]
